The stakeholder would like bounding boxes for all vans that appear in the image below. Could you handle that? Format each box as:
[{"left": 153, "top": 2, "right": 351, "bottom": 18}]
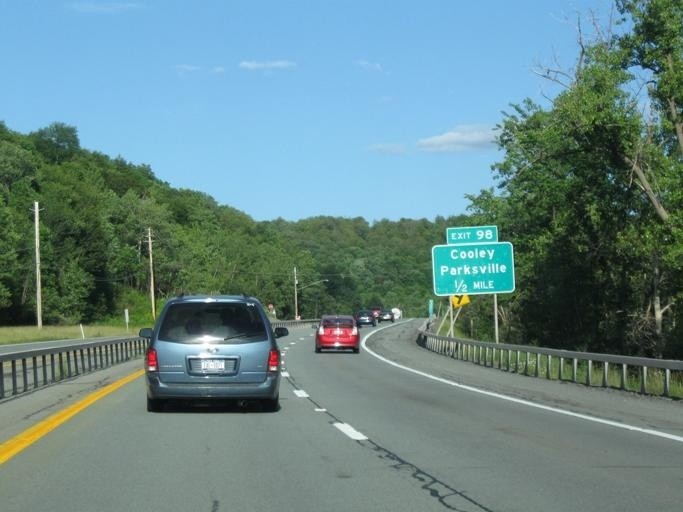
[{"left": 138, "top": 291, "right": 289, "bottom": 411}]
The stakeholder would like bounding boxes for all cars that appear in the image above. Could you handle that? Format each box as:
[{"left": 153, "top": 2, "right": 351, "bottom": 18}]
[
  {"left": 314, "top": 312, "right": 360, "bottom": 356},
  {"left": 356, "top": 306, "right": 401, "bottom": 325}
]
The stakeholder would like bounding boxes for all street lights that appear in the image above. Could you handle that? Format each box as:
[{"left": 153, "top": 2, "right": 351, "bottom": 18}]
[{"left": 293, "top": 278, "right": 330, "bottom": 319}]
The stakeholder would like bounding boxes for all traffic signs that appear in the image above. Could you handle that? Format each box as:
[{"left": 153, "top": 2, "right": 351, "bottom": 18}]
[
  {"left": 431, "top": 244, "right": 514, "bottom": 293},
  {"left": 445, "top": 226, "right": 497, "bottom": 241}
]
